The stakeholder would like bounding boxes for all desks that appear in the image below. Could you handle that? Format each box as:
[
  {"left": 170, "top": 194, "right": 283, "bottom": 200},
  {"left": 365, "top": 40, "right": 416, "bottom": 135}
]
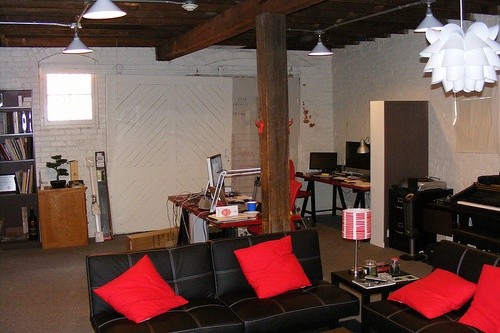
[
  {"left": 295, "top": 175, "right": 371, "bottom": 227},
  {"left": 168, "top": 195, "right": 301, "bottom": 246}
]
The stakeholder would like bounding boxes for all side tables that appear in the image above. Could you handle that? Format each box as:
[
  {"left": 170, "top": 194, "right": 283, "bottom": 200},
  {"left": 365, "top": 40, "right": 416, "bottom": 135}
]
[{"left": 331, "top": 267, "right": 420, "bottom": 333}]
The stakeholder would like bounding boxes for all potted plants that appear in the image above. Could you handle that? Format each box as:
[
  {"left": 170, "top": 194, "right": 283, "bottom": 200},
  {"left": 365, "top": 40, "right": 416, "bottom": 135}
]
[{"left": 46, "top": 155, "right": 68, "bottom": 188}]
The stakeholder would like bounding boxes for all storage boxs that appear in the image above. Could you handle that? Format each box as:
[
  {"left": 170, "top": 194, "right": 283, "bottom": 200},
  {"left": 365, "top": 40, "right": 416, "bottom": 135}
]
[{"left": 125, "top": 228, "right": 180, "bottom": 251}]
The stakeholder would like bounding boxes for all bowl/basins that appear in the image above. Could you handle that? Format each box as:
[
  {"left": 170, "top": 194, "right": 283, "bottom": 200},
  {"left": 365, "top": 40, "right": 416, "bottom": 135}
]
[{"left": 244, "top": 212, "right": 260, "bottom": 218}]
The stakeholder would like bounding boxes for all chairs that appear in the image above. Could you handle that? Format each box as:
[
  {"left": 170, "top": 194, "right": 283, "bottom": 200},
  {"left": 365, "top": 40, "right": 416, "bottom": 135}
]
[{"left": 288, "top": 159, "right": 311, "bottom": 230}]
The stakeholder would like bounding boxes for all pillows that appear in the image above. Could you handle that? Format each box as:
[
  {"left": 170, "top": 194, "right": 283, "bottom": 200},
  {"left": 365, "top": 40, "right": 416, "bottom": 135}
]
[
  {"left": 387, "top": 268, "right": 477, "bottom": 320},
  {"left": 233, "top": 235, "right": 312, "bottom": 300},
  {"left": 93, "top": 255, "right": 189, "bottom": 324},
  {"left": 457, "top": 263, "right": 500, "bottom": 332}
]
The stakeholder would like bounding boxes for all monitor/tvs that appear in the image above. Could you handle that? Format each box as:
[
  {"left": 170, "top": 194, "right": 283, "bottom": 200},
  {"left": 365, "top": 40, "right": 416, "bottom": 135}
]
[
  {"left": 345, "top": 141, "right": 370, "bottom": 176},
  {"left": 310, "top": 152, "right": 337, "bottom": 173},
  {"left": 204, "top": 154, "right": 224, "bottom": 197}
]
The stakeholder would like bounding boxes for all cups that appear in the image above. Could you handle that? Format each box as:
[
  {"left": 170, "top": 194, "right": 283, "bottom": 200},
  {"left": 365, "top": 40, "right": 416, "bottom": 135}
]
[{"left": 247, "top": 202, "right": 257, "bottom": 212}]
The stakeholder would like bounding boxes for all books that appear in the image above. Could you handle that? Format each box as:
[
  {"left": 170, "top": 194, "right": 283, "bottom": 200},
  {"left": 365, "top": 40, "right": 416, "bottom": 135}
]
[
  {"left": 23, "top": 97, "right": 31, "bottom": 102},
  {"left": 0, "top": 93, "right": 3, "bottom": 106},
  {"left": 15, "top": 165, "right": 34, "bottom": 194},
  {"left": 23, "top": 102, "right": 30, "bottom": 106},
  {"left": 0, "top": 136, "right": 31, "bottom": 160},
  {"left": 18, "top": 96, "right": 22, "bottom": 107},
  {"left": 0, "top": 111, "right": 32, "bottom": 133},
  {"left": 0, "top": 207, "right": 29, "bottom": 242}
]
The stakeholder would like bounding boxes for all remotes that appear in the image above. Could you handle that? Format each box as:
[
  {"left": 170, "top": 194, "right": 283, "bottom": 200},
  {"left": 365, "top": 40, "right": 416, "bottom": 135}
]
[{"left": 364, "top": 275, "right": 389, "bottom": 281}]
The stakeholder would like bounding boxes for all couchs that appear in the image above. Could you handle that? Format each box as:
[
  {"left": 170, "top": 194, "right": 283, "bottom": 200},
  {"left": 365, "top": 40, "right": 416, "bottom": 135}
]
[
  {"left": 86, "top": 229, "right": 360, "bottom": 332},
  {"left": 362, "top": 240, "right": 500, "bottom": 333}
]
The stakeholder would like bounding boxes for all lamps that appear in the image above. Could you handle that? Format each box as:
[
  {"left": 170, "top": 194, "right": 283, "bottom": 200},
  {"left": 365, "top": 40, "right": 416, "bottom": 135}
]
[
  {"left": 419, "top": 0, "right": 500, "bottom": 93},
  {"left": 356, "top": 137, "right": 370, "bottom": 154},
  {"left": 414, "top": 4, "right": 444, "bottom": 32},
  {"left": 62, "top": 29, "right": 94, "bottom": 54},
  {"left": 83, "top": 0, "right": 127, "bottom": 20},
  {"left": 308, "top": 35, "right": 333, "bottom": 56},
  {"left": 342, "top": 208, "right": 371, "bottom": 275}
]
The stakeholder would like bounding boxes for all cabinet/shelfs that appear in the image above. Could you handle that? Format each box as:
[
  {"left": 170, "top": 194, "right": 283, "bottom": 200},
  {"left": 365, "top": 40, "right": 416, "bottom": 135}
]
[
  {"left": 38, "top": 187, "right": 88, "bottom": 249},
  {"left": 0, "top": 89, "right": 40, "bottom": 249}
]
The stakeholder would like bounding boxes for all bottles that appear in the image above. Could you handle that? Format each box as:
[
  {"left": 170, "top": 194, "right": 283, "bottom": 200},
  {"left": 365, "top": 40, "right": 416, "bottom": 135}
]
[
  {"left": 389, "top": 257, "right": 401, "bottom": 276},
  {"left": 363, "top": 259, "right": 378, "bottom": 278},
  {"left": 28, "top": 208, "right": 38, "bottom": 240}
]
